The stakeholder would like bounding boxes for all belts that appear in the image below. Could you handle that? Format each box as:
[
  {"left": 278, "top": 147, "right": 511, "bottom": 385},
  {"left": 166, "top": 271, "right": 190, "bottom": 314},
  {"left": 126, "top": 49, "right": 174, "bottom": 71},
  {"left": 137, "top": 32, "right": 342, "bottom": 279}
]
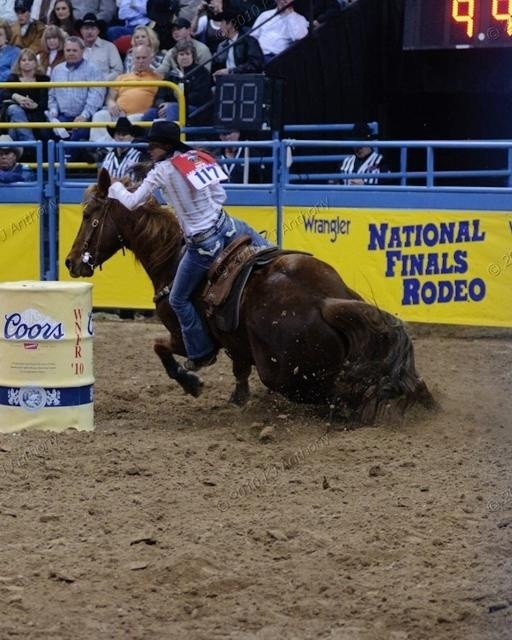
[{"left": 185, "top": 208, "right": 228, "bottom": 243}]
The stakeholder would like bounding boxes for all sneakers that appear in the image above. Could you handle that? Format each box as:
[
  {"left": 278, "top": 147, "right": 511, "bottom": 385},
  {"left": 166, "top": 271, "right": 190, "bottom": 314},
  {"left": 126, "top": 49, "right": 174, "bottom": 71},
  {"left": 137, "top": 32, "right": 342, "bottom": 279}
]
[{"left": 183, "top": 350, "right": 217, "bottom": 371}]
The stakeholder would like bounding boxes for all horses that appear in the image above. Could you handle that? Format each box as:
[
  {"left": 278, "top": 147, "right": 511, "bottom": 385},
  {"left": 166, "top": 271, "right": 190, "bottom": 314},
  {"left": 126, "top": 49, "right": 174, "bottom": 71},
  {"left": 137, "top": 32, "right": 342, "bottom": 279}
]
[{"left": 66, "top": 167, "right": 441, "bottom": 425}]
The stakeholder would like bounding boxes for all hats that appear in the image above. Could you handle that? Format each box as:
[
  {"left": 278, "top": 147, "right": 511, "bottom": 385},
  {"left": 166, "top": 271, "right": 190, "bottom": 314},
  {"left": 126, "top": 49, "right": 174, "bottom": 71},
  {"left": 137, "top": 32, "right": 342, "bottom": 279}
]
[
  {"left": 73, "top": 12, "right": 108, "bottom": 33},
  {"left": 340, "top": 121, "right": 381, "bottom": 141},
  {"left": 0, "top": 133, "right": 24, "bottom": 159},
  {"left": 131, "top": 120, "right": 194, "bottom": 152},
  {"left": 211, "top": 5, "right": 242, "bottom": 22},
  {"left": 105, "top": 116, "right": 147, "bottom": 139},
  {"left": 166, "top": 16, "right": 191, "bottom": 30},
  {"left": 13, "top": 0, "right": 34, "bottom": 14}
]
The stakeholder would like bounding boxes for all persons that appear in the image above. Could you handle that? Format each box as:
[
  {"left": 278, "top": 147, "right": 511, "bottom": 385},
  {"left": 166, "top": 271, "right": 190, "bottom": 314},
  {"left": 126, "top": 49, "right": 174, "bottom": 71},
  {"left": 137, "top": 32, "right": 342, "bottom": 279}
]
[
  {"left": 292, "top": 0, "right": 341, "bottom": 29},
  {"left": 142, "top": 41, "right": 215, "bottom": 125},
  {"left": 104, "top": 0, "right": 157, "bottom": 43},
  {"left": 210, "top": 6, "right": 264, "bottom": 97},
  {"left": 210, "top": 121, "right": 261, "bottom": 185},
  {"left": 82, "top": 44, "right": 166, "bottom": 162},
  {"left": 124, "top": 25, "right": 166, "bottom": 76},
  {"left": 157, "top": 18, "right": 212, "bottom": 74},
  {"left": 340, "top": 120, "right": 389, "bottom": 187},
  {"left": 0, "top": 1, "right": 42, "bottom": 26},
  {"left": 146, "top": 0, "right": 225, "bottom": 49},
  {"left": 35, "top": 36, "right": 106, "bottom": 162},
  {"left": 247, "top": 0, "right": 310, "bottom": 65},
  {"left": 0, "top": 48, "right": 50, "bottom": 161},
  {"left": 73, "top": 12, "right": 123, "bottom": 82},
  {"left": 11, "top": 1, "right": 46, "bottom": 55},
  {"left": 0, "top": 134, "right": 36, "bottom": 182},
  {"left": 47, "top": 1, "right": 115, "bottom": 29},
  {"left": 106, "top": 122, "right": 276, "bottom": 371},
  {"left": 36, "top": 26, "right": 68, "bottom": 78},
  {"left": 50, "top": 1, "right": 80, "bottom": 37},
  {"left": 0, "top": 17, "right": 22, "bottom": 82},
  {"left": 99, "top": 116, "right": 149, "bottom": 192},
  {"left": 207, "top": 0, "right": 276, "bottom": 37}
]
